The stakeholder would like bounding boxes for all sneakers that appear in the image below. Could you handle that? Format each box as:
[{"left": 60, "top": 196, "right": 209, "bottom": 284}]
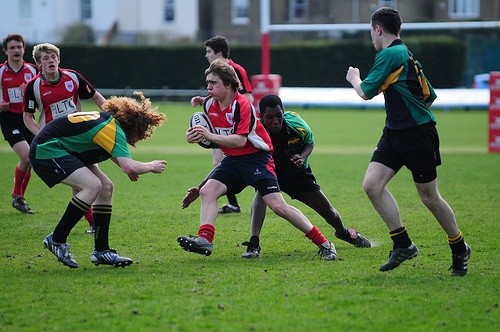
[
  {"left": 448, "top": 241, "right": 471, "bottom": 276},
  {"left": 12, "top": 197, "right": 35, "bottom": 213},
  {"left": 90, "top": 249, "right": 133, "bottom": 268},
  {"left": 241, "top": 241, "right": 261, "bottom": 257},
  {"left": 218, "top": 203, "right": 240, "bottom": 214},
  {"left": 316, "top": 241, "right": 337, "bottom": 260},
  {"left": 176, "top": 234, "right": 214, "bottom": 256},
  {"left": 43, "top": 232, "right": 79, "bottom": 268},
  {"left": 335, "top": 227, "right": 370, "bottom": 248},
  {"left": 378, "top": 242, "right": 419, "bottom": 272}
]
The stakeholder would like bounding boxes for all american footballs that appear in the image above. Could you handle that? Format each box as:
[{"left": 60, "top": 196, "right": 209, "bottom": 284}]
[{"left": 190, "top": 113, "right": 215, "bottom": 150}]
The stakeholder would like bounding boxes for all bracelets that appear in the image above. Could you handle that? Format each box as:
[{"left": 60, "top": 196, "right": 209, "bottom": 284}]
[{"left": 199, "top": 99, "right": 203, "bottom": 107}]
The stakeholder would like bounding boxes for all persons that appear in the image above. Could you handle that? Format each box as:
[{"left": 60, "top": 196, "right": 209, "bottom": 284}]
[
  {"left": 176, "top": 56, "right": 338, "bottom": 262},
  {"left": 19, "top": 42, "right": 115, "bottom": 234},
  {"left": 27, "top": 90, "right": 168, "bottom": 236},
  {"left": 344, "top": 5, "right": 472, "bottom": 279},
  {"left": 0, "top": 33, "right": 41, "bottom": 215},
  {"left": 180, "top": 93, "right": 373, "bottom": 260},
  {"left": 189, "top": 33, "right": 273, "bottom": 214}
]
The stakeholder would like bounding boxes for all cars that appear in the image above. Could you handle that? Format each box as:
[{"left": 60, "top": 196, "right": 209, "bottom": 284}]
[{"left": 471, "top": 73, "right": 491, "bottom": 110}]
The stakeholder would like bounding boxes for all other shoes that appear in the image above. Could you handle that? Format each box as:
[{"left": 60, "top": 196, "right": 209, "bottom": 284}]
[{"left": 87, "top": 225, "right": 95, "bottom": 233}]
